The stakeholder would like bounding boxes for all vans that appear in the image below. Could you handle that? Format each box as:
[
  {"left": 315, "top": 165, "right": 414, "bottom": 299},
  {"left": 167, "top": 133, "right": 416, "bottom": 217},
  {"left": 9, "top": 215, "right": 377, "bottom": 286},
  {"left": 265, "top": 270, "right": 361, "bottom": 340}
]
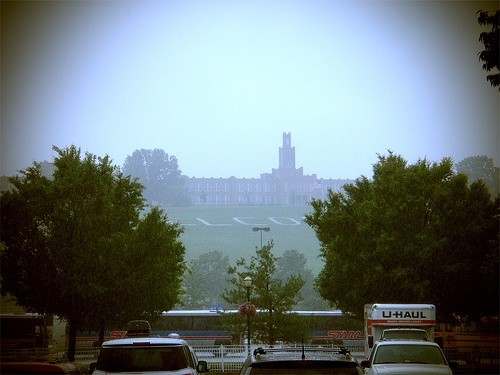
[{"left": 0, "top": 313, "right": 51, "bottom": 356}]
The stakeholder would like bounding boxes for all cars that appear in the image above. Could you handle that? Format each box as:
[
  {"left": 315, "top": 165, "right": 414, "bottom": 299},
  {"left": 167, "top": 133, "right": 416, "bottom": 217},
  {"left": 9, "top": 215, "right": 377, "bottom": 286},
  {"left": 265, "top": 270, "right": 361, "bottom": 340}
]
[
  {"left": 238, "top": 347, "right": 363, "bottom": 375},
  {"left": 361, "top": 339, "right": 453, "bottom": 375},
  {"left": 89, "top": 338, "right": 208, "bottom": 375}
]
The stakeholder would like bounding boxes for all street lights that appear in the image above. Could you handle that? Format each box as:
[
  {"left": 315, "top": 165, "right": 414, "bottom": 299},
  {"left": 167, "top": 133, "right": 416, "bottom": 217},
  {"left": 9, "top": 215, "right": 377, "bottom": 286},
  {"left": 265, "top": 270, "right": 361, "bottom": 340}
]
[
  {"left": 243, "top": 276, "right": 252, "bottom": 355},
  {"left": 253, "top": 227, "right": 271, "bottom": 249}
]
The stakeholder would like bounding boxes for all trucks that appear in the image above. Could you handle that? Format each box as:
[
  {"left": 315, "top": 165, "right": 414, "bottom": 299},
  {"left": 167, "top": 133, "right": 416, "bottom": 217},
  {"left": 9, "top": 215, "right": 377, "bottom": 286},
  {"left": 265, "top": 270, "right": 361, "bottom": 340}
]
[{"left": 363, "top": 303, "right": 436, "bottom": 356}]
